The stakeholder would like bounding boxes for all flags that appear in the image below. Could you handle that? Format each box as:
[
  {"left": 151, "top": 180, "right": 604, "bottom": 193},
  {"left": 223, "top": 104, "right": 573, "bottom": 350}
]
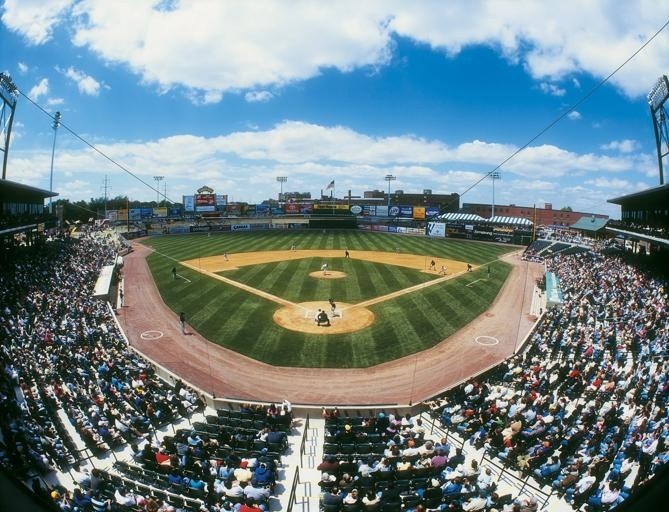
[{"left": 324, "top": 180, "right": 335, "bottom": 191}]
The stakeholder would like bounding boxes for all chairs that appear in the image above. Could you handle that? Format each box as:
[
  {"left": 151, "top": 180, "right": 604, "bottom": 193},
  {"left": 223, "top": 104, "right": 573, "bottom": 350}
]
[
  {"left": 0, "top": 230, "right": 207, "bottom": 479},
  {"left": 426, "top": 232, "right": 669, "bottom": 512},
  {"left": 28, "top": 388, "right": 557, "bottom": 512}
]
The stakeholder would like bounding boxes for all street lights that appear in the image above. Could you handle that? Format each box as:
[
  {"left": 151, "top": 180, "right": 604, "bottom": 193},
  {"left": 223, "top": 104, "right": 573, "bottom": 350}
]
[
  {"left": 48, "top": 110, "right": 61, "bottom": 216},
  {"left": 154, "top": 175, "right": 165, "bottom": 208},
  {"left": 276, "top": 176, "right": 287, "bottom": 204},
  {"left": 488, "top": 170, "right": 501, "bottom": 221},
  {"left": 0, "top": 70, "right": 20, "bottom": 181},
  {"left": 385, "top": 174, "right": 396, "bottom": 206},
  {"left": 648, "top": 74, "right": 668, "bottom": 186}
]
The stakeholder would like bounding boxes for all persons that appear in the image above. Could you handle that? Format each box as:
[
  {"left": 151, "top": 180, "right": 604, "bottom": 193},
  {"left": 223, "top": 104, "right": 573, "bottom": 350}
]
[
  {"left": 345, "top": 250, "right": 349, "bottom": 257},
  {"left": 131, "top": 347, "right": 523, "bottom": 512},
  {"left": 291, "top": 244, "right": 295, "bottom": 250},
  {"left": 440, "top": 265, "right": 446, "bottom": 274},
  {"left": 430, "top": 260, "right": 435, "bottom": 269},
  {"left": 180, "top": 313, "right": 185, "bottom": 335},
  {"left": 223, "top": 252, "right": 226, "bottom": 260},
  {"left": 315, "top": 298, "right": 336, "bottom": 326},
  {"left": 322, "top": 263, "right": 328, "bottom": 273},
  {"left": 522, "top": 214, "right": 668, "bottom": 512},
  {"left": 467, "top": 264, "right": 472, "bottom": 272},
  {"left": 172, "top": 265, "right": 177, "bottom": 279},
  {"left": 2, "top": 214, "right": 131, "bottom": 512}
]
